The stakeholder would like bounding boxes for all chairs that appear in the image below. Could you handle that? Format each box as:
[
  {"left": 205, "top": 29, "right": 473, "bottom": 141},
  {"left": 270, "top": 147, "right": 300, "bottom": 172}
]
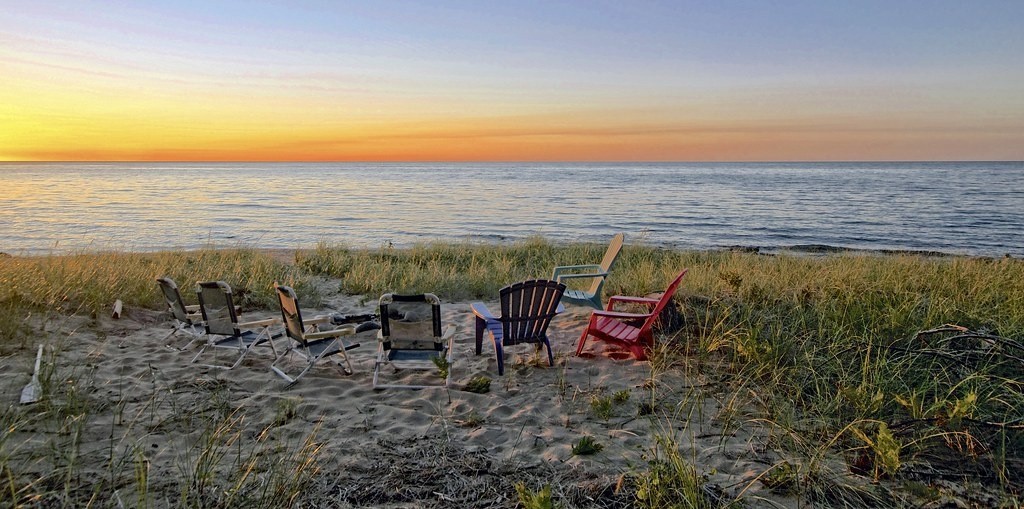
[
  {"left": 550, "top": 232, "right": 625, "bottom": 311},
  {"left": 372, "top": 293, "right": 455, "bottom": 390},
  {"left": 470, "top": 278, "right": 566, "bottom": 376},
  {"left": 271, "top": 284, "right": 360, "bottom": 388},
  {"left": 156, "top": 278, "right": 242, "bottom": 353},
  {"left": 191, "top": 281, "right": 281, "bottom": 371},
  {"left": 576, "top": 267, "right": 688, "bottom": 361}
]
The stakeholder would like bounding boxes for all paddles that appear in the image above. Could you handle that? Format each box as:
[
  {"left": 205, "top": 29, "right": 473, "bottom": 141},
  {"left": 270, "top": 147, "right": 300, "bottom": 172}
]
[{"left": 19, "top": 343, "right": 44, "bottom": 404}]
